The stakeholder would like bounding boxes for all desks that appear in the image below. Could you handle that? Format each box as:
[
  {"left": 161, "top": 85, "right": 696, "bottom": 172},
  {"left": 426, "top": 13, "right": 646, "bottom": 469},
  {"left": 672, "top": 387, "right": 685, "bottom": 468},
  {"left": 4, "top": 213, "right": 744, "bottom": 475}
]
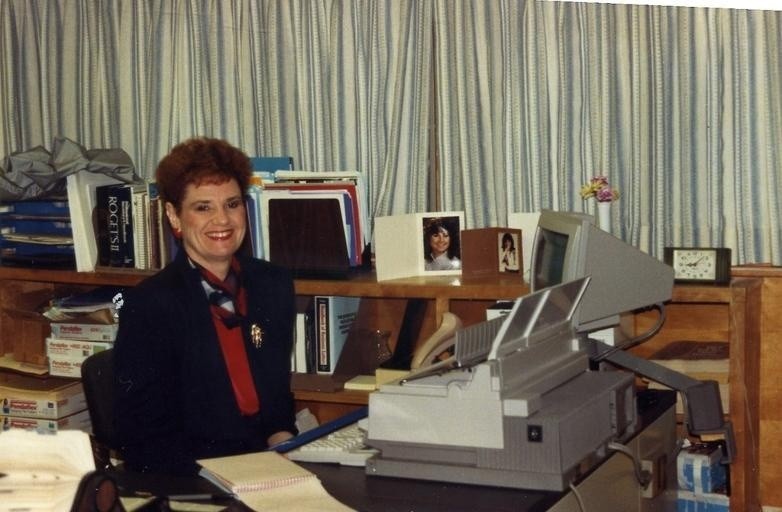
[{"left": 134, "top": 387, "right": 681, "bottom": 511}]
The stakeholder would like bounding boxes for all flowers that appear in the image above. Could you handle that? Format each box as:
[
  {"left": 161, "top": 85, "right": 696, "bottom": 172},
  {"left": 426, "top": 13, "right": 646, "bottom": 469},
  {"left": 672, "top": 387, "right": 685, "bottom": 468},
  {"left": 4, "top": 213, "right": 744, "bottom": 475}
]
[{"left": 579, "top": 176, "right": 620, "bottom": 202}]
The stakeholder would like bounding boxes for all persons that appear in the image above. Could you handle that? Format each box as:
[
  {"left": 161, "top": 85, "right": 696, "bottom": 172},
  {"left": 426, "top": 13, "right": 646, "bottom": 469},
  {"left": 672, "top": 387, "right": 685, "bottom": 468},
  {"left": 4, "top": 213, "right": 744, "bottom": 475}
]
[
  {"left": 498, "top": 233, "right": 519, "bottom": 273},
  {"left": 113, "top": 138, "right": 298, "bottom": 476},
  {"left": 423, "top": 217, "right": 461, "bottom": 270}
]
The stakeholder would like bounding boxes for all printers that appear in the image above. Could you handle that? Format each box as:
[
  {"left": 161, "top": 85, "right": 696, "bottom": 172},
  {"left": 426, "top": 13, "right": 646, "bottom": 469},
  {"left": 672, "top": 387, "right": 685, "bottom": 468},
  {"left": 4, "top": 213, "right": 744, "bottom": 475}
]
[{"left": 366, "top": 274, "right": 639, "bottom": 492}]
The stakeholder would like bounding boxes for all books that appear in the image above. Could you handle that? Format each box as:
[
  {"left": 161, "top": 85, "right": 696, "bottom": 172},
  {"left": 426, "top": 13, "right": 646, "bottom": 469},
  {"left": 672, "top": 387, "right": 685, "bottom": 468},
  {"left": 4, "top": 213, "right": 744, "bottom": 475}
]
[
  {"left": 44, "top": 295, "right": 119, "bottom": 378},
  {"left": 344, "top": 375, "right": 375, "bottom": 390},
  {"left": 196, "top": 451, "right": 358, "bottom": 512},
  {"left": 245, "top": 157, "right": 368, "bottom": 278},
  {"left": 0, "top": 380, "right": 97, "bottom": 512},
  {"left": 0, "top": 170, "right": 171, "bottom": 272},
  {"left": 290, "top": 296, "right": 360, "bottom": 376}
]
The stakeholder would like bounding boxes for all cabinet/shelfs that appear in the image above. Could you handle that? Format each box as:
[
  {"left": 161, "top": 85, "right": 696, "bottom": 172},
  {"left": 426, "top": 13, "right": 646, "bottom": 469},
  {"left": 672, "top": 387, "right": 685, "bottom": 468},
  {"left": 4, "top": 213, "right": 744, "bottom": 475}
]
[{"left": 1, "top": 256, "right": 762, "bottom": 512}]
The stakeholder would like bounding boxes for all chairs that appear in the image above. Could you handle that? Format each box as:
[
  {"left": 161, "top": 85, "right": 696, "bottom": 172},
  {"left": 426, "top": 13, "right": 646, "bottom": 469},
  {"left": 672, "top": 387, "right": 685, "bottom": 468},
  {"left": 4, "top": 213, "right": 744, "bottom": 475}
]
[{"left": 82, "top": 346, "right": 117, "bottom": 471}]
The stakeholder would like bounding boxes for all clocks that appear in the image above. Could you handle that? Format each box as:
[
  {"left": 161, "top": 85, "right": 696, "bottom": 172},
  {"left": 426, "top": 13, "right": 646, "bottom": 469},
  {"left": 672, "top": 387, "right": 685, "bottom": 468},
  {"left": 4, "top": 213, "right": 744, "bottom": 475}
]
[{"left": 664, "top": 246, "right": 732, "bottom": 283}]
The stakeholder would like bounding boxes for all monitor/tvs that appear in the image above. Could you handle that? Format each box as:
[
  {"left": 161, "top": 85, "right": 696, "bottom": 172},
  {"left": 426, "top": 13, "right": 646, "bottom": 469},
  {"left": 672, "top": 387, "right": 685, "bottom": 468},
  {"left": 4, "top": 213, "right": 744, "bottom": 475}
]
[{"left": 528, "top": 209, "right": 674, "bottom": 333}]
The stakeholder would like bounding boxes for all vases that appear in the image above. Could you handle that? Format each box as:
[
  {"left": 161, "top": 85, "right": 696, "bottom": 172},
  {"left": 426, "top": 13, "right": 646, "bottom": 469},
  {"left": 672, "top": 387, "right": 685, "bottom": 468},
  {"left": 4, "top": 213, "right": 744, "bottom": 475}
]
[{"left": 598, "top": 203, "right": 611, "bottom": 233}]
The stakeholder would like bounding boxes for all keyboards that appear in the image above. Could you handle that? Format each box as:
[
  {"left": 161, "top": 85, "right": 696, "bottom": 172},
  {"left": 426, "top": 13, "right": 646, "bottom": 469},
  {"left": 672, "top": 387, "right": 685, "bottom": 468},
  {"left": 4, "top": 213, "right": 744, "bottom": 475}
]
[{"left": 283, "top": 416, "right": 380, "bottom": 466}]
